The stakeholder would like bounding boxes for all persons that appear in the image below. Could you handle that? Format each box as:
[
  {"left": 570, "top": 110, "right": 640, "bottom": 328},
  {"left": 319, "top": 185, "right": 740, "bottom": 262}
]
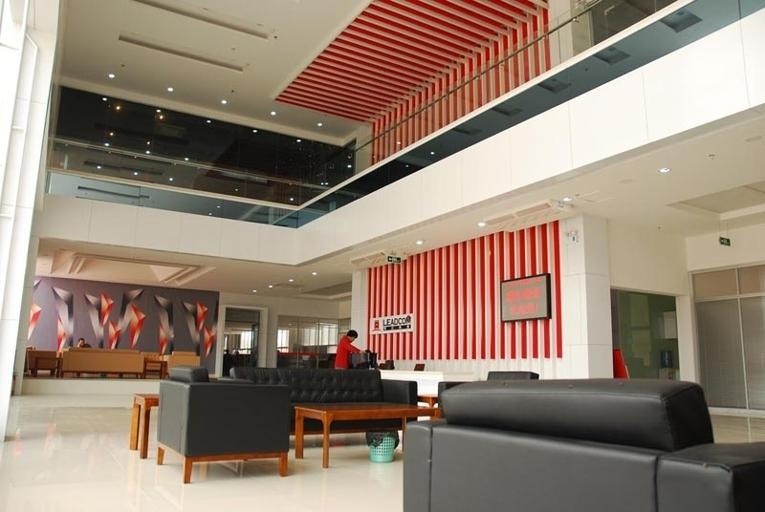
[
  {"left": 77, "top": 338, "right": 91, "bottom": 348},
  {"left": 335, "top": 331, "right": 366, "bottom": 369},
  {"left": 222, "top": 350, "right": 233, "bottom": 376}
]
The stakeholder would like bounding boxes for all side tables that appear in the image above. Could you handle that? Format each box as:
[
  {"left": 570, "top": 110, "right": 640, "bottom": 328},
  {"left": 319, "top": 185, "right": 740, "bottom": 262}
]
[
  {"left": 128, "top": 392, "right": 161, "bottom": 458},
  {"left": 415, "top": 393, "right": 439, "bottom": 408}
]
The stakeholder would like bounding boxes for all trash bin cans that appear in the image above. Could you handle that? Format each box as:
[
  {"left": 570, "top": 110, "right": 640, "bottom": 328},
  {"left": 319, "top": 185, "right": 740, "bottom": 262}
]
[{"left": 369, "top": 431, "right": 397, "bottom": 463}]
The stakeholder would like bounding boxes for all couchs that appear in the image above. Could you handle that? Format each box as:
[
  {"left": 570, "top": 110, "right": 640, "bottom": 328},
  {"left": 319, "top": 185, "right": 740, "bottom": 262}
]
[
  {"left": 216, "top": 364, "right": 417, "bottom": 435},
  {"left": 402, "top": 377, "right": 765, "bottom": 512},
  {"left": 61, "top": 347, "right": 145, "bottom": 379}
]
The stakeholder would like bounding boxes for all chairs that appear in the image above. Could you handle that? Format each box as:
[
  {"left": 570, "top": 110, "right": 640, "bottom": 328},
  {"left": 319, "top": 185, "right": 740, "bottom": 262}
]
[
  {"left": 140, "top": 351, "right": 166, "bottom": 379},
  {"left": 161, "top": 350, "right": 202, "bottom": 378},
  {"left": 26, "top": 346, "right": 62, "bottom": 378},
  {"left": 155, "top": 365, "right": 292, "bottom": 484},
  {"left": 436, "top": 370, "right": 539, "bottom": 423}
]
